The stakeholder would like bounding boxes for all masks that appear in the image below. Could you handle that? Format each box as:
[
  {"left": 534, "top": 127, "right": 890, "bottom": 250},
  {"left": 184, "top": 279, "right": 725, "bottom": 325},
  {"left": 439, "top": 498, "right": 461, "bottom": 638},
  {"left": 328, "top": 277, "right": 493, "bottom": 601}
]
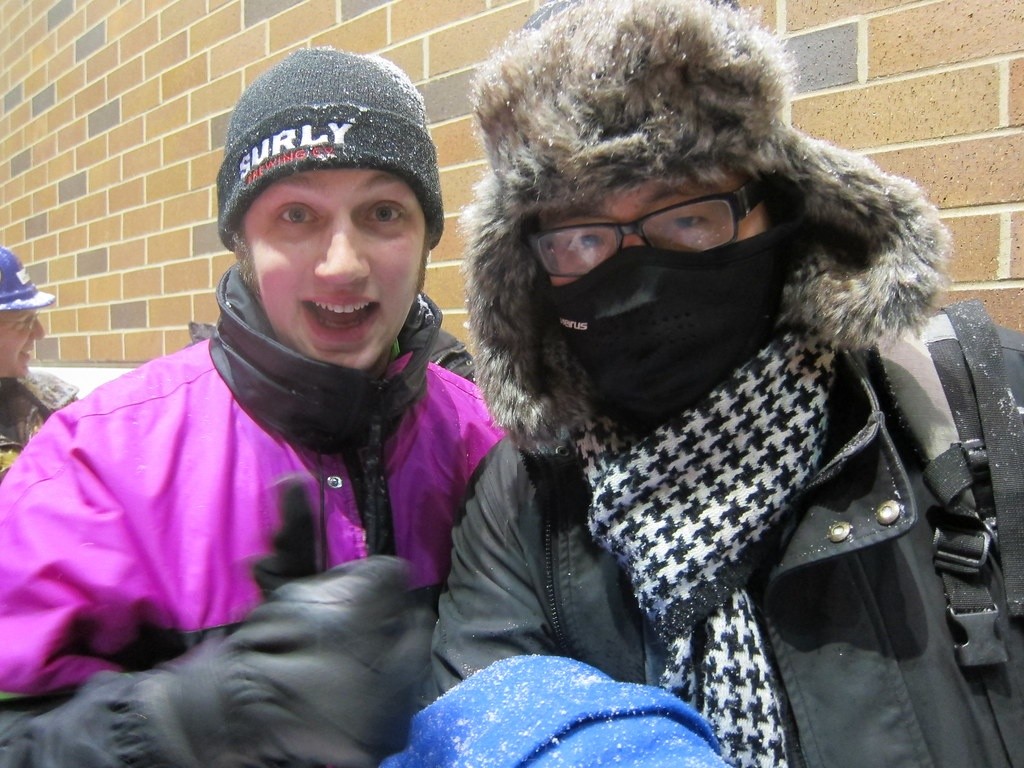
[{"left": 536, "top": 226, "right": 798, "bottom": 437}]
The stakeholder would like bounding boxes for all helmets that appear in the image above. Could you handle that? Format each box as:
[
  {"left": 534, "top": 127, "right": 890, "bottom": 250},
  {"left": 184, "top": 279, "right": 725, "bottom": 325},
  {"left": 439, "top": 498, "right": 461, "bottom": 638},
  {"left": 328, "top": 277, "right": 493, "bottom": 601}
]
[{"left": 0, "top": 248, "right": 55, "bottom": 311}]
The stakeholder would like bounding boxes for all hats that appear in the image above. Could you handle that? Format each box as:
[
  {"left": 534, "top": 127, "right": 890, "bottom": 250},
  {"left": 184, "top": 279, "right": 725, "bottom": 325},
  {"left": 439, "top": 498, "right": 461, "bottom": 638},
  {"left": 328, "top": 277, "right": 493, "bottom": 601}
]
[
  {"left": 214, "top": 46, "right": 445, "bottom": 253},
  {"left": 456, "top": 0, "right": 953, "bottom": 456}
]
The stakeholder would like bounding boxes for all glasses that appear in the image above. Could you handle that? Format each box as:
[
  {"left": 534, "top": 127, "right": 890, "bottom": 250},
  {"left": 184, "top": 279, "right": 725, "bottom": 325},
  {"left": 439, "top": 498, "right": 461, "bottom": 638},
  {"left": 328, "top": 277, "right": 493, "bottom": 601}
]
[
  {"left": 532, "top": 180, "right": 770, "bottom": 280},
  {"left": 0, "top": 311, "right": 42, "bottom": 330}
]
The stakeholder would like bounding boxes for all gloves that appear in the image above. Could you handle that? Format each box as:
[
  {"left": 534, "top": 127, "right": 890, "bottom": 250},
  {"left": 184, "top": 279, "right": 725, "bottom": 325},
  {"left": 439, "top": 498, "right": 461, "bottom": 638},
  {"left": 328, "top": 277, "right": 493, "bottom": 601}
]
[{"left": 35, "top": 562, "right": 432, "bottom": 768}]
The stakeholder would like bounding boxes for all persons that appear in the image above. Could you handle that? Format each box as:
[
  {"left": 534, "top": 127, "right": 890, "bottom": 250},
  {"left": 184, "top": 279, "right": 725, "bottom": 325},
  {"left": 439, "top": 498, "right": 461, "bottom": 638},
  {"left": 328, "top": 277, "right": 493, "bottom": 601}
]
[
  {"left": 0, "top": 247, "right": 80, "bottom": 487},
  {"left": 0, "top": 47, "right": 509, "bottom": 768},
  {"left": 426, "top": 0, "right": 1024, "bottom": 768}
]
